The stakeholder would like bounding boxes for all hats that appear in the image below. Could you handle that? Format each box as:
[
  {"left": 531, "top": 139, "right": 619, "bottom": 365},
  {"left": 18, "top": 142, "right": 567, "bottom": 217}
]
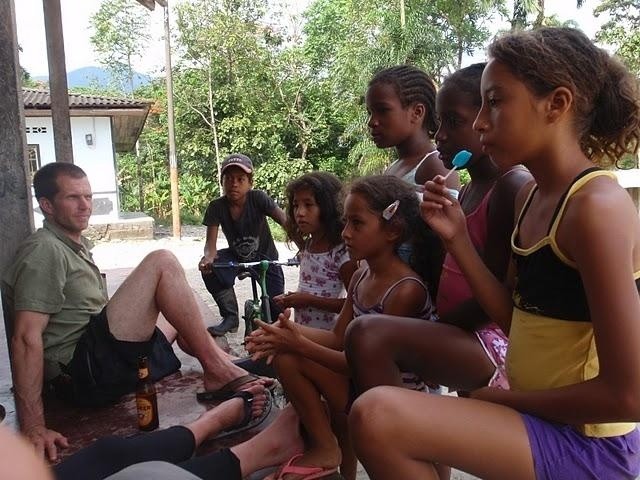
[{"left": 221, "top": 154, "right": 254, "bottom": 173}]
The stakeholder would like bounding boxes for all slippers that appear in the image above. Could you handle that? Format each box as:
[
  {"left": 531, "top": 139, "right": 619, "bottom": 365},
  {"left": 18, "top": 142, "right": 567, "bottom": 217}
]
[
  {"left": 262, "top": 454, "right": 338, "bottom": 480},
  {"left": 196, "top": 372, "right": 280, "bottom": 443}
]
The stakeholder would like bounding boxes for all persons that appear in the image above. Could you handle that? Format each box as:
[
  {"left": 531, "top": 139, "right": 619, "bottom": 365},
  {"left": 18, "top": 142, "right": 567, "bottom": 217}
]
[
  {"left": 286, "top": 174, "right": 359, "bottom": 330},
  {"left": 367, "top": 63, "right": 459, "bottom": 294},
  {"left": 200, "top": 154, "right": 306, "bottom": 337},
  {"left": 344, "top": 64, "right": 531, "bottom": 396},
  {"left": 1, "top": 162, "right": 278, "bottom": 465},
  {"left": 350, "top": 27, "right": 640, "bottom": 480},
  {"left": 244, "top": 175, "right": 432, "bottom": 480},
  {"left": 0, "top": 384, "right": 331, "bottom": 480}
]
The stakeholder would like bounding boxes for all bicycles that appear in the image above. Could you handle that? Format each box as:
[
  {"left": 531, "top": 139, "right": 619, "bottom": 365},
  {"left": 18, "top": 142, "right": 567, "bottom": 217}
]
[{"left": 205, "top": 259, "right": 300, "bottom": 355}]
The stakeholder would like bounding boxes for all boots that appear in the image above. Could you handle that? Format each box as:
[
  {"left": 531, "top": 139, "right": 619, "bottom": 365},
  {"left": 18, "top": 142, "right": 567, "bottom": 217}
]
[{"left": 207, "top": 287, "right": 238, "bottom": 336}]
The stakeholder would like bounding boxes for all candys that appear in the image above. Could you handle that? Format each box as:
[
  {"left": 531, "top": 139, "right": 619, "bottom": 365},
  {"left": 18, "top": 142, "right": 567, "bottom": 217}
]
[{"left": 444, "top": 150, "right": 473, "bottom": 182}]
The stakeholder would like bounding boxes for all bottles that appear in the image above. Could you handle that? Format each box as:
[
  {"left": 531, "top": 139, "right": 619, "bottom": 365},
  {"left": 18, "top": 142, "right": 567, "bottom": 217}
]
[{"left": 135, "top": 355, "right": 159, "bottom": 432}]
